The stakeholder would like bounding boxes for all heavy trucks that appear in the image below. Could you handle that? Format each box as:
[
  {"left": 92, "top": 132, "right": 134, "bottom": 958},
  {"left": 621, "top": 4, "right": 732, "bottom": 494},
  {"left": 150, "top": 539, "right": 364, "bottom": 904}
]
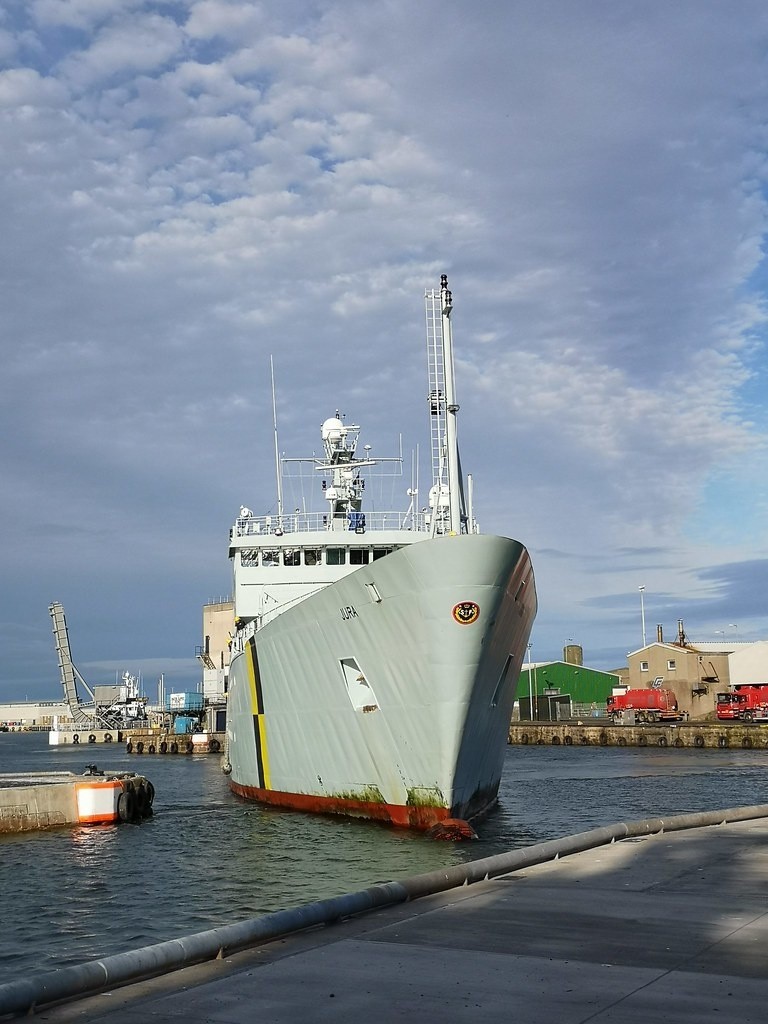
[
  {"left": 606, "top": 689, "right": 690, "bottom": 724},
  {"left": 714, "top": 685, "right": 768, "bottom": 723}
]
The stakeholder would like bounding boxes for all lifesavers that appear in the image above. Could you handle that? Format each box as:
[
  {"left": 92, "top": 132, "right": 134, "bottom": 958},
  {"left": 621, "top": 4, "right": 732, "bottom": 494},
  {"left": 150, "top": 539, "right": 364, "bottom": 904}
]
[
  {"left": 618, "top": 737, "right": 626, "bottom": 746},
  {"left": 719, "top": 737, "right": 728, "bottom": 747},
  {"left": 127, "top": 743, "right": 133, "bottom": 751},
  {"left": 658, "top": 737, "right": 667, "bottom": 747},
  {"left": 186, "top": 742, "right": 194, "bottom": 750},
  {"left": 674, "top": 738, "right": 683, "bottom": 747},
  {"left": 137, "top": 742, "right": 144, "bottom": 751},
  {"left": 522, "top": 734, "right": 528, "bottom": 743},
  {"left": 104, "top": 734, "right": 112, "bottom": 740},
  {"left": 507, "top": 735, "right": 512, "bottom": 744},
  {"left": 209, "top": 739, "right": 221, "bottom": 751},
  {"left": 89, "top": 735, "right": 96, "bottom": 741},
  {"left": 564, "top": 736, "right": 572, "bottom": 745},
  {"left": 73, "top": 734, "right": 78, "bottom": 740},
  {"left": 552, "top": 736, "right": 560, "bottom": 745},
  {"left": 171, "top": 743, "right": 178, "bottom": 753},
  {"left": 600, "top": 733, "right": 607, "bottom": 745},
  {"left": 149, "top": 746, "right": 155, "bottom": 754},
  {"left": 694, "top": 736, "right": 704, "bottom": 747},
  {"left": 638, "top": 736, "right": 647, "bottom": 746},
  {"left": 161, "top": 742, "right": 168, "bottom": 752},
  {"left": 742, "top": 737, "right": 752, "bottom": 748},
  {"left": 537, "top": 739, "right": 544, "bottom": 745}
]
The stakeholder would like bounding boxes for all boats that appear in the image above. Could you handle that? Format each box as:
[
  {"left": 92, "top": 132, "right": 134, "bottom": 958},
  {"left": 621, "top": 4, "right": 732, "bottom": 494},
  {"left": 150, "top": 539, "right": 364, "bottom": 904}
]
[{"left": 222, "top": 272, "right": 537, "bottom": 831}]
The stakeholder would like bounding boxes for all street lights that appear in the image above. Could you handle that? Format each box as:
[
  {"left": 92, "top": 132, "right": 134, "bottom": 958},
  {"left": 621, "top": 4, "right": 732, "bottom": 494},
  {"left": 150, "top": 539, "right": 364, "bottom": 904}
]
[
  {"left": 729, "top": 624, "right": 738, "bottom": 643},
  {"left": 565, "top": 638, "right": 574, "bottom": 663},
  {"left": 527, "top": 643, "right": 534, "bottom": 721},
  {"left": 639, "top": 585, "right": 647, "bottom": 647},
  {"left": 714, "top": 630, "right": 726, "bottom": 642}
]
